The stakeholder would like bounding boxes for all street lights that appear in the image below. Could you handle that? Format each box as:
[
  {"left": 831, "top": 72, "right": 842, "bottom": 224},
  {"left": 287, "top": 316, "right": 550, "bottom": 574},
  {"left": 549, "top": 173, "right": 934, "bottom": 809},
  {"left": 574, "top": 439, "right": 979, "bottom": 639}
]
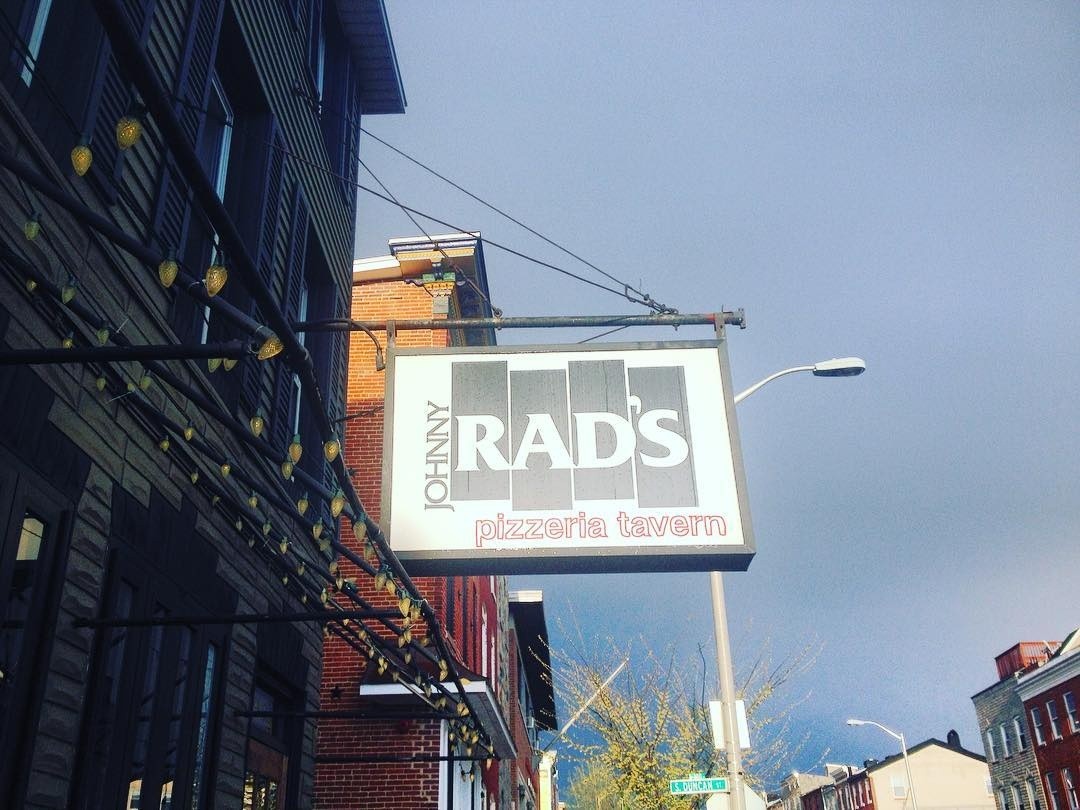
[
  {"left": 701, "top": 357, "right": 871, "bottom": 810},
  {"left": 846, "top": 719, "right": 918, "bottom": 810}
]
